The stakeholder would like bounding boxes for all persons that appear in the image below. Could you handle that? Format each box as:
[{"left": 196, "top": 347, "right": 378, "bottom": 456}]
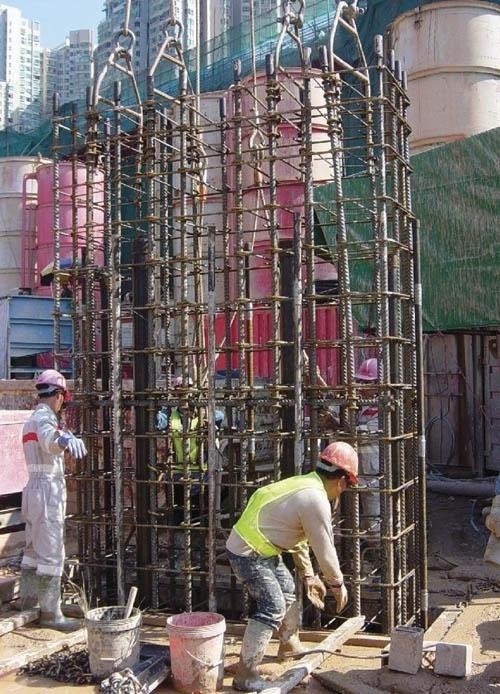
[
  {"left": 19, "top": 368, "right": 87, "bottom": 629},
  {"left": 225, "top": 442, "right": 359, "bottom": 693},
  {"left": 161, "top": 376, "right": 202, "bottom": 521}
]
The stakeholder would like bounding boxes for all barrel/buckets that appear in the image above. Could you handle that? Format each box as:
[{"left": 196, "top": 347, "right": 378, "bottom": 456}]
[
  {"left": 84, "top": 605, "right": 144, "bottom": 683},
  {"left": 166, "top": 610, "right": 227, "bottom": 694}
]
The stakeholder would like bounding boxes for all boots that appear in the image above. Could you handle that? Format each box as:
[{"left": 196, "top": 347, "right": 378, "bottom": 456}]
[
  {"left": 231, "top": 618, "right": 271, "bottom": 693},
  {"left": 276, "top": 603, "right": 309, "bottom": 663},
  {"left": 36, "top": 574, "right": 83, "bottom": 632},
  {"left": 15, "top": 567, "right": 39, "bottom": 612}
]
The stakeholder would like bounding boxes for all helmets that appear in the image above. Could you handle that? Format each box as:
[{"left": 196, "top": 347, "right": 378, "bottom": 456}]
[
  {"left": 173, "top": 376, "right": 194, "bottom": 388},
  {"left": 315, "top": 442, "right": 359, "bottom": 486},
  {"left": 35, "top": 369, "right": 68, "bottom": 396},
  {"left": 354, "top": 356, "right": 378, "bottom": 382}
]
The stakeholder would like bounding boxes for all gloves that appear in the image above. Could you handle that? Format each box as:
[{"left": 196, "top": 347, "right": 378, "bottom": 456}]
[
  {"left": 329, "top": 581, "right": 349, "bottom": 615},
  {"left": 59, "top": 433, "right": 88, "bottom": 459},
  {"left": 303, "top": 574, "right": 327, "bottom": 611}
]
